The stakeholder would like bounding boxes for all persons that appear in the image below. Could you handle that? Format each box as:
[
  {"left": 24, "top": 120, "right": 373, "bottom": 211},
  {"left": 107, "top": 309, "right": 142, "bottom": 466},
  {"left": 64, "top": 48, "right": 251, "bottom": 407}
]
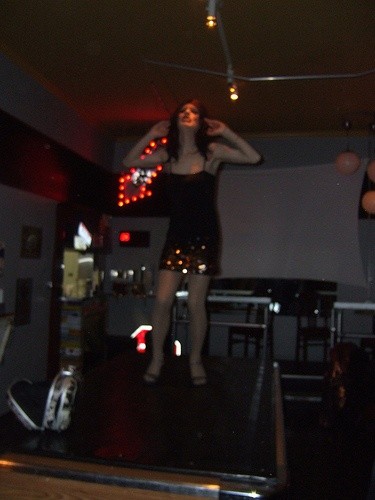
[
  {"left": 285, "top": 340, "right": 375, "bottom": 500},
  {"left": 121, "top": 97, "right": 262, "bottom": 388}
]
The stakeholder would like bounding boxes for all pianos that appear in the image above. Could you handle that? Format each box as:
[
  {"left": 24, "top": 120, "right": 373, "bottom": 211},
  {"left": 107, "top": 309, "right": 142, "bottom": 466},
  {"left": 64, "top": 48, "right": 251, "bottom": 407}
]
[{"left": 169, "top": 278, "right": 339, "bottom": 369}]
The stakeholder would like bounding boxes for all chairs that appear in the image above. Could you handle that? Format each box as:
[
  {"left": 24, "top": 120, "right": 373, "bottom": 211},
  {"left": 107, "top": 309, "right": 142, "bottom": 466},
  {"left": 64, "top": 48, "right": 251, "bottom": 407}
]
[
  {"left": 295, "top": 290, "right": 332, "bottom": 364},
  {"left": 227, "top": 287, "right": 276, "bottom": 358}
]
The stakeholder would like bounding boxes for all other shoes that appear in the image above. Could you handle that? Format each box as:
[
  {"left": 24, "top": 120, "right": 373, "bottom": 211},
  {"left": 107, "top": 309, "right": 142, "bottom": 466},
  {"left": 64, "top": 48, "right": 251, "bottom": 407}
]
[
  {"left": 190, "top": 362, "right": 207, "bottom": 386},
  {"left": 143, "top": 368, "right": 162, "bottom": 386}
]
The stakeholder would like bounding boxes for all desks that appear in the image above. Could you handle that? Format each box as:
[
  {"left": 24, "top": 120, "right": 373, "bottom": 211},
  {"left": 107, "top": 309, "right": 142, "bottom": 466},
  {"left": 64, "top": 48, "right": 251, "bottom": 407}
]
[{"left": 333, "top": 301, "right": 375, "bottom": 348}]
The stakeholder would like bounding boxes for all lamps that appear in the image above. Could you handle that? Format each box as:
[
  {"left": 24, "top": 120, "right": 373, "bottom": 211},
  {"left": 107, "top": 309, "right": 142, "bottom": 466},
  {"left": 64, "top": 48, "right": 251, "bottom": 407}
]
[
  {"left": 335, "top": 121, "right": 375, "bottom": 216},
  {"left": 206, "top": 0, "right": 238, "bottom": 104}
]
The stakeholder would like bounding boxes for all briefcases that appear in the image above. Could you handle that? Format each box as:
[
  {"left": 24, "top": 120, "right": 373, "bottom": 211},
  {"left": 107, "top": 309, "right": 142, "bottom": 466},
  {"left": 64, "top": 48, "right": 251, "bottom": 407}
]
[{"left": 6, "top": 365, "right": 81, "bottom": 431}]
